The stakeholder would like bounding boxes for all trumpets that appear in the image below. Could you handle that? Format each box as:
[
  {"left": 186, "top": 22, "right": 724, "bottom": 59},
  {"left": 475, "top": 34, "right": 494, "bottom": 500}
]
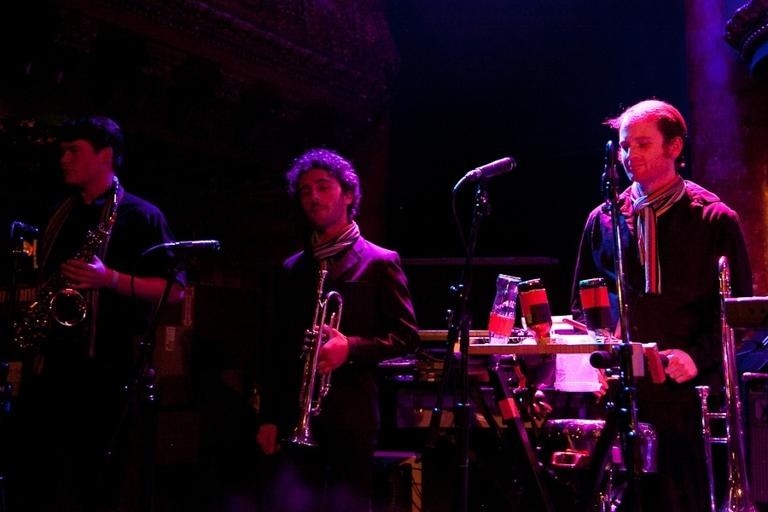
[{"left": 281, "top": 270, "right": 344, "bottom": 447}]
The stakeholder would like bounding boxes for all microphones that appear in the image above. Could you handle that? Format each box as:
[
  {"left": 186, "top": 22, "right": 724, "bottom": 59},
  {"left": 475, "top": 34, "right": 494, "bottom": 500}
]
[
  {"left": 599, "top": 140, "right": 621, "bottom": 192},
  {"left": 461, "top": 158, "right": 519, "bottom": 181},
  {"left": 172, "top": 237, "right": 221, "bottom": 255}
]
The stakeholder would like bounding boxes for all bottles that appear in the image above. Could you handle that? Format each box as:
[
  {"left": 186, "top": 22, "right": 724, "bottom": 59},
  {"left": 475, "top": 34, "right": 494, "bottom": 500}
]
[
  {"left": 489, "top": 275, "right": 553, "bottom": 341},
  {"left": 578, "top": 277, "right": 614, "bottom": 332}
]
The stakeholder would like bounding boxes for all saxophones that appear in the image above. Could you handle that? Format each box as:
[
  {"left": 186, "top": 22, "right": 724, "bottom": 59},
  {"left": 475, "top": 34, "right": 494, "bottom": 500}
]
[{"left": 13, "top": 176, "right": 119, "bottom": 349}]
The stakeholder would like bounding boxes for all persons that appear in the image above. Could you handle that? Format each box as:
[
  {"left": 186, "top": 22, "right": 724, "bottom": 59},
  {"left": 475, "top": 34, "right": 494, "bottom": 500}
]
[
  {"left": 8, "top": 116, "right": 186, "bottom": 512},
  {"left": 570, "top": 97, "right": 755, "bottom": 512},
  {"left": 253, "top": 145, "right": 420, "bottom": 512}
]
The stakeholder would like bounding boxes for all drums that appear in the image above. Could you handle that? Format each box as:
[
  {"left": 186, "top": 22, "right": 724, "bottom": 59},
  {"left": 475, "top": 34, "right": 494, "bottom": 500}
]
[{"left": 543, "top": 419, "right": 658, "bottom": 474}]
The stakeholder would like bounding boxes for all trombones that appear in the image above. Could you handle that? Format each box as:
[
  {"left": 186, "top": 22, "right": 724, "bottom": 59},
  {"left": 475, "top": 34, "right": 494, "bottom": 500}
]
[{"left": 695, "top": 255, "right": 758, "bottom": 512}]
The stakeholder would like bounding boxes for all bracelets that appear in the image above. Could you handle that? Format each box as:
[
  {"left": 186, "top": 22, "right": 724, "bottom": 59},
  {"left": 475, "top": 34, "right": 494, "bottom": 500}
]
[
  {"left": 130, "top": 273, "right": 136, "bottom": 297},
  {"left": 111, "top": 269, "right": 119, "bottom": 291}
]
